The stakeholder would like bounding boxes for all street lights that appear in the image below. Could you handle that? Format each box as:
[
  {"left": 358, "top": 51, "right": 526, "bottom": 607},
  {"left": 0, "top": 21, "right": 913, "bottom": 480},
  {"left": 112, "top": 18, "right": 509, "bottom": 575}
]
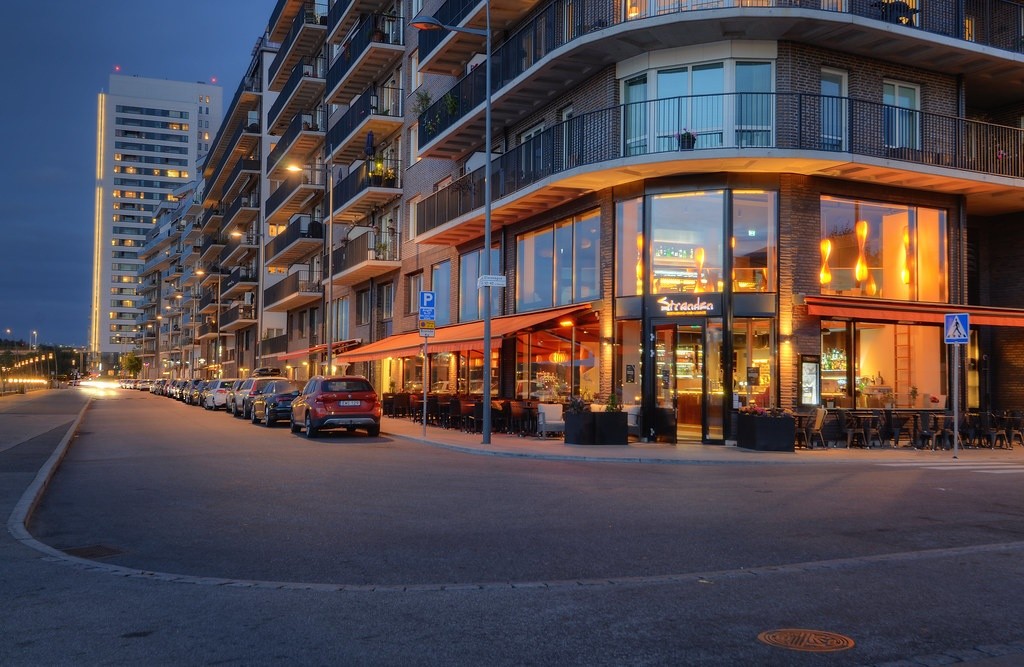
[
  {"left": 230, "top": 215, "right": 264, "bottom": 368},
  {"left": 409, "top": 0, "right": 494, "bottom": 446},
  {"left": 132, "top": 329, "right": 146, "bottom": 380},
  {"left": 195, "top": 258, "right": 221, "bottom": 379},
  {"left": 157, "top": 315, "right": 174, "bottom": 379},
  {"left": 148, "top": 324, "right": 160, "bottom": 379},
  {"left": 175, "top": 284, "right": 195, "bottom": 380},
  {"left": 33, "top": 330, "right": 37, "bottom": 349}
]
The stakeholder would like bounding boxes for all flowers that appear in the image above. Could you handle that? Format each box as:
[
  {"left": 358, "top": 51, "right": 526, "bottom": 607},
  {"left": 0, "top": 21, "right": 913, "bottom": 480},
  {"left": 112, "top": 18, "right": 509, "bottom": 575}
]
[
  {"left": 737, "top": 403, "right": 795, "bottom": 417},
  {"left": 674, "top": 127, "right": 697, "bottom": 141},
  {"left": 567, "top": 393, "right": 623, "bottom": 414}
]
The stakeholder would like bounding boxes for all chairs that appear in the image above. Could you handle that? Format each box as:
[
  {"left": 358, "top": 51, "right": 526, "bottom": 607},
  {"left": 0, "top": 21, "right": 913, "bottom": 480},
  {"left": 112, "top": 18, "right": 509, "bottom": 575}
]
[
  {"left": 382, "top": 392, "right": 569, "bottom": 439},
  {"left": 535, "top": 403, "right": 565, "bottom": 440},
  {"left": 795, "top": 406, "right": 1024, "bottom": 451}
]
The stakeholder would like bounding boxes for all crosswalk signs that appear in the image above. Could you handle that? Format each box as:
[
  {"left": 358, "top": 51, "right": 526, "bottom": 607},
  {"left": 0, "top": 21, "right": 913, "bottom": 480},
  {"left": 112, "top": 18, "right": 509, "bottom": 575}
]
[{"left": 944, "top": 313, "right": 969, "bottom": 344}]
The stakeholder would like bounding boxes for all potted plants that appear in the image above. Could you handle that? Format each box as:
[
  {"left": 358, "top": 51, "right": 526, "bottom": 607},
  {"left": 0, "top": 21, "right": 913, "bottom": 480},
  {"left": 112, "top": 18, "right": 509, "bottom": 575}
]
[
  {"left": 375, "top": 242, "right": 388, "bottom": 260},
  {"left": 371, "top": 170, "right": 383, "bottom": 187},
  {"left": 384, "top": 169, "right": 395, "bottom": 188}
]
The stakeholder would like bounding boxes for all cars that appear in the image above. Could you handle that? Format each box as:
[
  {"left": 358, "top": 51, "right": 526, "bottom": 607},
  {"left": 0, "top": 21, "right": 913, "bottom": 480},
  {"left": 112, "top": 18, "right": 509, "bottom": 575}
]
[
  {"left": 250, "top": 379, "right": 308, "bottom": 427},
  {"left": 119, "top": 378, "right": 245, "bottom": 414}
]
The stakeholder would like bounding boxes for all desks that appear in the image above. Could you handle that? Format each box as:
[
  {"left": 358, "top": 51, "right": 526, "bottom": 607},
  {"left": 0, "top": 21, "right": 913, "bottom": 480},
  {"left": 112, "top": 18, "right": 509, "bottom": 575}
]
[
  {"left": 853, "top": 415, "right": 879, "bottom": 449},
  {"left": 916, "top": 413, "right": 955, "bottom": 450},
  {"left": 414, "top": 400, "right": 428, "bottom": 425},
  {"left": 465, "top": 404, "right": 478, "bottom": 433},
  {"left": 996, "top": 415, "right": 1021, "bottom": 448},
  {"left": 386, "top": 395, "right": 396, "bottom": 419},
  {"left": 437, "top": 401, "right": 451, "bottom": 430},
  {"left": 793, "top": 414, "right": 816, "bottom": 449},
  {"left": 517, "top": 405, "right": 538, "bottom": 437}
]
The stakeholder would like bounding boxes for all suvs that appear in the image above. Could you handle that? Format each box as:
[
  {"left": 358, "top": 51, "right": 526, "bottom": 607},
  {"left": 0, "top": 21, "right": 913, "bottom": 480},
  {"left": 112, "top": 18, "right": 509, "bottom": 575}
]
[
  {"left": 242, "top": 376, "right": 287, "bottom": 420},
  {"left": 289, "top": 374, "right": 381, "bottom": 438}
]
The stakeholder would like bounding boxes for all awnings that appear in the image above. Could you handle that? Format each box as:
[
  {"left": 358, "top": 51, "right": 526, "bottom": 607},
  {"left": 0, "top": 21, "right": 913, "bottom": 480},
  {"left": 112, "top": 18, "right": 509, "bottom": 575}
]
[
  {"left": 277, "top": 339, "right": 357, "bottom": 361},
  {"left": 335, "top": 306, "right": 593, "bottom": 365},
  {"left": 805, "top": 305, "right": 1024, "bottom": 328}
]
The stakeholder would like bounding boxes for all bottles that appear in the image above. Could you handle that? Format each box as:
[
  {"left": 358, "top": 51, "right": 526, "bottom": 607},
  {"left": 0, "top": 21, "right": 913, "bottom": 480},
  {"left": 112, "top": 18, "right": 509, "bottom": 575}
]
[
  {"left": 823, "top": 346, "right": 847, "bottom": 369},
  {"left": 870, "top": 371, "right": 883, "bottom": 386},
  {"left": 655, "top": 245, "right": 694, "bottom": 259}
]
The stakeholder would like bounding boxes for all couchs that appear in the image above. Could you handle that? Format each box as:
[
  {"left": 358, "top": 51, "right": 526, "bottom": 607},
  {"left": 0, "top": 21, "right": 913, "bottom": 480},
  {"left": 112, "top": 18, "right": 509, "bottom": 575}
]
[{"left": 589, "top": 403, "right": 642, "bottom": 442}]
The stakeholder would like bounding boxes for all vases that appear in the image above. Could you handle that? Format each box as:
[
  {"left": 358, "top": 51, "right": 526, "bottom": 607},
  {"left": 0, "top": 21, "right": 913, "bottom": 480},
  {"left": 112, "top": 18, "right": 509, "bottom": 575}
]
[
  {"left": 737, "top": 416, "right": 796, "bottom": 453},
  {"left": 564, "top": 411, "right": 628, "bottom": 446},
  {"left": 678, "top": 137, "right": 694, "bottom": 151}
]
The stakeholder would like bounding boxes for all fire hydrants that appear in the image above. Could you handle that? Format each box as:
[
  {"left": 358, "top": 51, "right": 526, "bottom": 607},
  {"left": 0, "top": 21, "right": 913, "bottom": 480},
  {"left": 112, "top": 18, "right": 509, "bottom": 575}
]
[{"left": 287, "top": 144, "right": 334, "bottom": 377}]
